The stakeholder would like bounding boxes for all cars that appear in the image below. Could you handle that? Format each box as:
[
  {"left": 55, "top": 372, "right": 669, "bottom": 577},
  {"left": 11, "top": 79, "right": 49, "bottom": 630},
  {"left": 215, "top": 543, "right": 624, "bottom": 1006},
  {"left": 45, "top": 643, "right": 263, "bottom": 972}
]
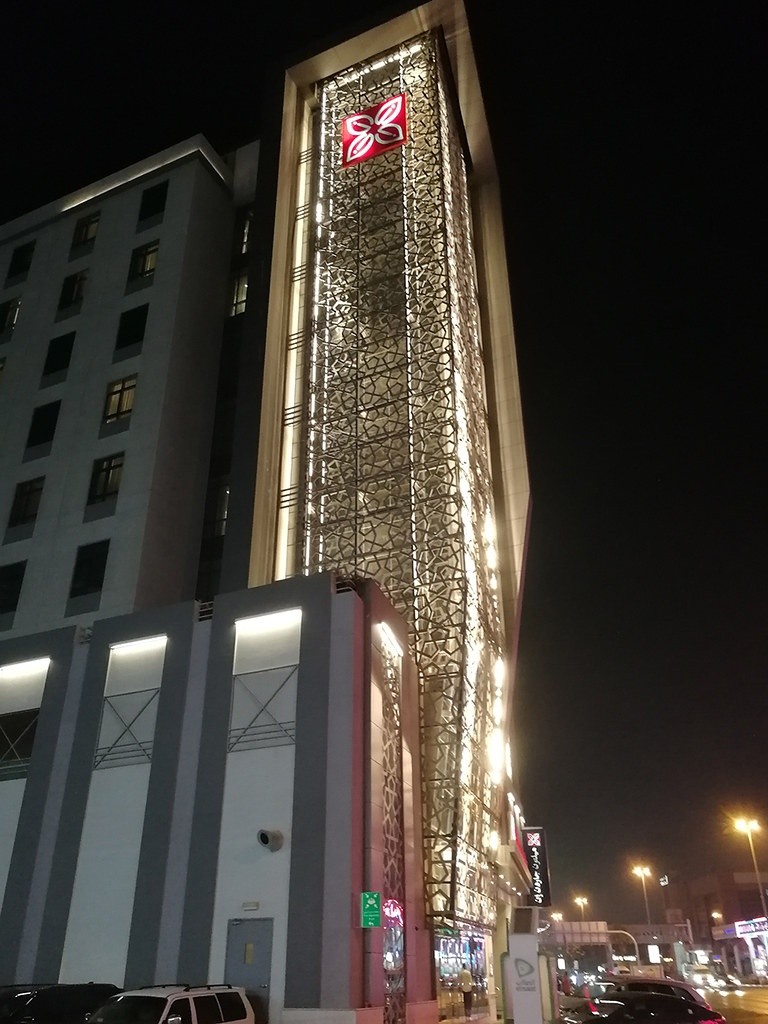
[
  {"left": 714, "top": 974, "right": 742, "bottom": 990},
  {"left": 550, "top": 975, "right": 727, "bottom": 1024}
]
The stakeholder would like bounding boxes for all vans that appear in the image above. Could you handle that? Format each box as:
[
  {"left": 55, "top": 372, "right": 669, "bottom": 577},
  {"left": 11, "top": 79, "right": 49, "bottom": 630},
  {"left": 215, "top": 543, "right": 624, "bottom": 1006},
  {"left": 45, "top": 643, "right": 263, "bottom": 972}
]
[{"left": 683, "top": 963, "right": 711, "bottom": 986}]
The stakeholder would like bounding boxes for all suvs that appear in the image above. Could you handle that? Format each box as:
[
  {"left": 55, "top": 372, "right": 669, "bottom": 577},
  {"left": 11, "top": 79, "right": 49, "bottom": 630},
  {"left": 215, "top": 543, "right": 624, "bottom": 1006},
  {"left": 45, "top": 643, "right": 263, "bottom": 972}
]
[{"left": 0, "top": 979, "right": 256, "bottom": 1024}]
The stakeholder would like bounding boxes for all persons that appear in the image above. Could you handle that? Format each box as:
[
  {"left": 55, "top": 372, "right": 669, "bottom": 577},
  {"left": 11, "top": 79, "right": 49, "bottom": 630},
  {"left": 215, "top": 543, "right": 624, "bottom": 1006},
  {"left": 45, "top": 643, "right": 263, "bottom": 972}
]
[
  {"left": 562, "top": 971, "right": 571, "bottom": 995},
  {"left": 460, "top": 963, "right": 473, "bottom": 1018}
]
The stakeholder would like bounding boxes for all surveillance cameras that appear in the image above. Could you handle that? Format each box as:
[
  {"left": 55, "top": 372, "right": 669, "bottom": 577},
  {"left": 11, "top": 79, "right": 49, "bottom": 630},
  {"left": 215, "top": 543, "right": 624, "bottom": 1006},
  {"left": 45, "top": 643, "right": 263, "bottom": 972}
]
[{"left": 258, "top": 829, "right": 283, "bottom": 852}]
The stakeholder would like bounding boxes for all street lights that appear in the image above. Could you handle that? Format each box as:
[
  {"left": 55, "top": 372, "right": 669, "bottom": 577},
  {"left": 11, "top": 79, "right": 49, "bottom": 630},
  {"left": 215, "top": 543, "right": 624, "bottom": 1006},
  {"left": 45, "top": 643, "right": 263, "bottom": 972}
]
[
  {"left": 574, "top": 898, "right": 588, "bottom": 922},
  {"left": 632, "top": 865, "right": 651, "bottom": 924},
  {"left": 733, "top": 818, "right": 768, "bottom": 916}
]
